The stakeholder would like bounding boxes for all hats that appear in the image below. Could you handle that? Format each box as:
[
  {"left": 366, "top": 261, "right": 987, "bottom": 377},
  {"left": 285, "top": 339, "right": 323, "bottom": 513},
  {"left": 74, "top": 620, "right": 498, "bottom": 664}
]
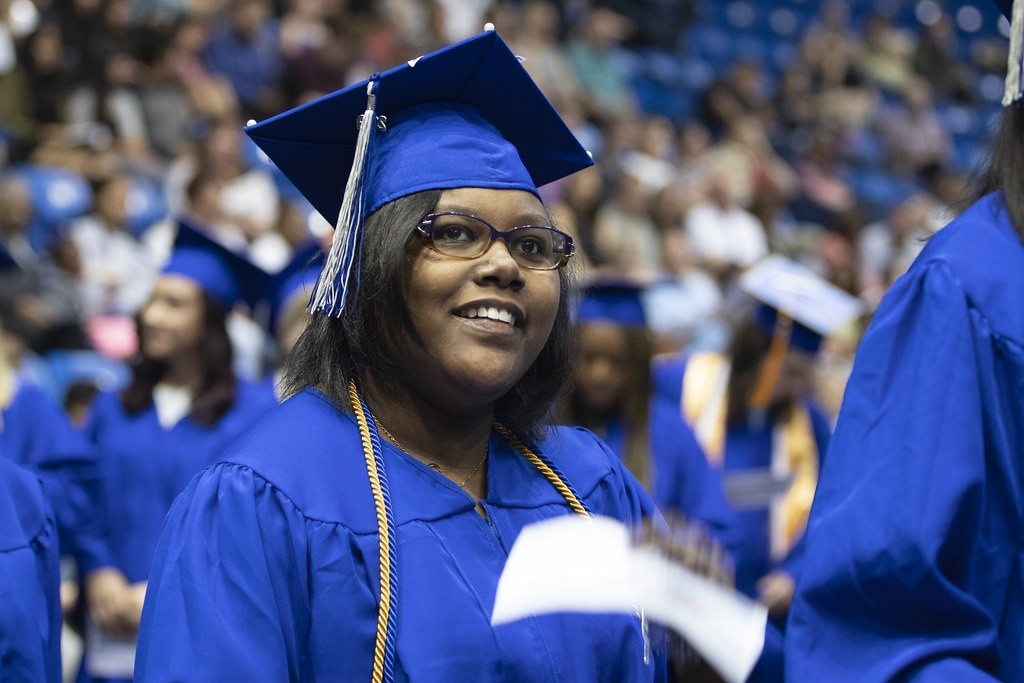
[
  {"left": 572, "top": 273, "right": 665, "bottom": 326},
  {"left": 160, "top": 215, "right": 268, "bottom": 312},
  {"left": 739, "top": 250, "right": 867, "bottom": 355},
  {"left": 242, "top": 27, "right": 594, "bottom": 233}
]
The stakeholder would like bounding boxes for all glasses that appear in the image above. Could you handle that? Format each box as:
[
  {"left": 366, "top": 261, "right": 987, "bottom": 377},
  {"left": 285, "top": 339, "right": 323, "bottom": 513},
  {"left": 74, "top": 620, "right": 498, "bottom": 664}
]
[{"left": 415, "top": 209, "right": 577, "bottom": 272}]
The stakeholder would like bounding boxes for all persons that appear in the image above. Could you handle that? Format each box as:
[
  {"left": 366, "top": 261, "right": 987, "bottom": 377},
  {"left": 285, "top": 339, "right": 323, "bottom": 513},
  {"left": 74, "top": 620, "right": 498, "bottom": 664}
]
[
  {"left": 784, "top": 0, "right": 1023, "bottom": 683},
  {"left": 0, "top": 292, "right": 80, "bottom": 622},
  {"left": 67, "top": 217, "right": 273, "bottom": 676},
  {"left": 1, "top": 0, "right": 1004, "bottom": 417},
  {"left": 650, "top": 256, "right": 865, "bottom": 623},
  {"left": 129, "top": 20, "right": 786, "bottom": 683},
  {"left": 3, "top": 447, "right": 65, "bottom": 683},
  {"left": 563, "top": 278, "right": 741, "bottom": 682}
]
